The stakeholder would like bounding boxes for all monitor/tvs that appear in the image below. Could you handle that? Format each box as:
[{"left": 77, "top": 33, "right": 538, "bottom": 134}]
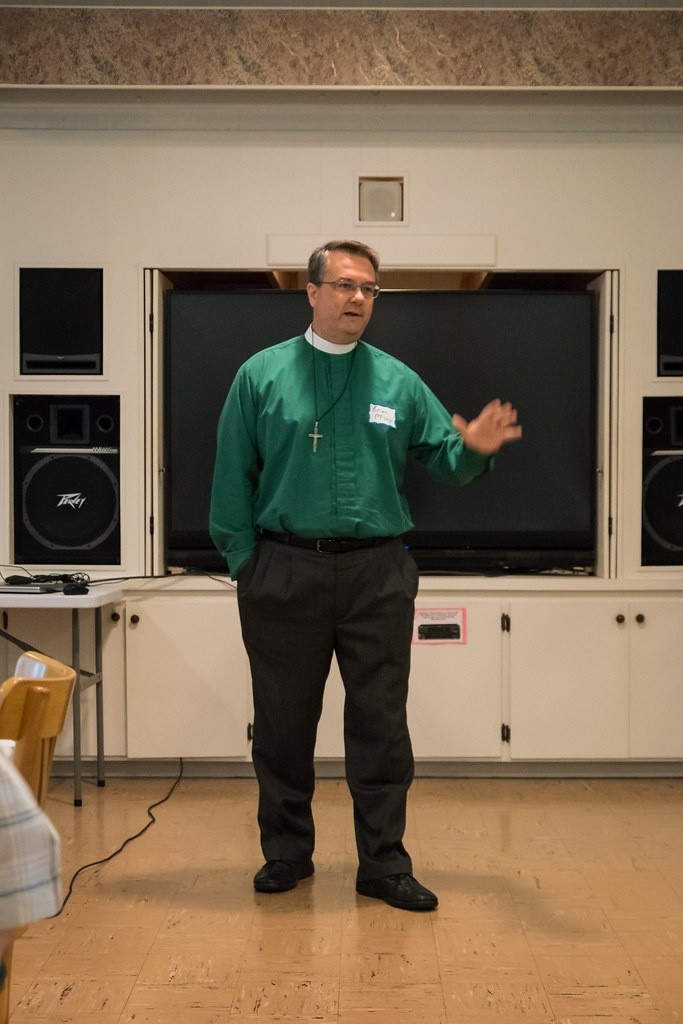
[{"left": 161, "top": 289, "right": 602, "bottom": 576}]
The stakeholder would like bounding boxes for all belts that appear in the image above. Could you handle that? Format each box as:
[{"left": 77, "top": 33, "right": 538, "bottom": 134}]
[{"left": 256, "top": 526, "right": 394, "bottom": 553}]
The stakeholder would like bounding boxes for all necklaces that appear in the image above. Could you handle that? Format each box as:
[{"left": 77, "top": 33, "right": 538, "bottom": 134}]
[{"left": 308, "top": 324, "right": 357, "bottom": 454}]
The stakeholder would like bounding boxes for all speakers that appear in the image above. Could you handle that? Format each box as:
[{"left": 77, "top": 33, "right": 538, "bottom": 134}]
[
  {"left": 657, "top": 269, "right": 683, "bottom": 377},
  {"left": 19, "top": 267, "right": 102, "bottom": 375},
  {"left": 642, "top": 396, "right": 683, "bottom": 566},
  {"left": 12, "top": 394, "right": 121, "bottom": 566}
]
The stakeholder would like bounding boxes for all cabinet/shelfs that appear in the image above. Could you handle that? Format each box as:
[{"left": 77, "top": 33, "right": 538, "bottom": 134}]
[{"left": 0, "top": 597, "right": 682, "bottom": 764}]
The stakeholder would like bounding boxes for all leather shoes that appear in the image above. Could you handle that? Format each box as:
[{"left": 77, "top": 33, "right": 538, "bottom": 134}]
[
  {"left": 253, "top": 859, "right": 315, "bottom": 892},
  {"left": 357, "top": 872, "right": 438, "bottom": 909}
]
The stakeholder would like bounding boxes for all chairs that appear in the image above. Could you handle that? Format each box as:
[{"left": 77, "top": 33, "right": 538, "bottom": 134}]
[{"left": 0, "top": 650, "right": 77, "bottom": 808}]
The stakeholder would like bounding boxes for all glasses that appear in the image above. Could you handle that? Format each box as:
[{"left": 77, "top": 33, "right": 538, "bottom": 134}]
[{"left": 316, "top": 279, "right": 380, "bottom": 299}]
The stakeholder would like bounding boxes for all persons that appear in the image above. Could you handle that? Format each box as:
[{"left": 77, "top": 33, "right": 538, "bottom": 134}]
[{"left": 209, "top": 241, "right": 522, "bottom": 911}]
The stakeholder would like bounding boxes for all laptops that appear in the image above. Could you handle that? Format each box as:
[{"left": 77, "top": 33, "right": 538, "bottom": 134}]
[{"left": 0, "top": 582, "right": 71, "bottom": 593}]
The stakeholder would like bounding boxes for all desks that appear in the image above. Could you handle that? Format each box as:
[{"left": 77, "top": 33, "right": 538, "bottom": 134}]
[{"left": 0, "top": 586, "right": 124, "bottom": 806}]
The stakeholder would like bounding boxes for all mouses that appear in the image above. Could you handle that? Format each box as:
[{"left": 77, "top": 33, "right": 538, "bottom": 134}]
[{"left": 63, "top": 584, "right": 89, "bottom": 595}]
